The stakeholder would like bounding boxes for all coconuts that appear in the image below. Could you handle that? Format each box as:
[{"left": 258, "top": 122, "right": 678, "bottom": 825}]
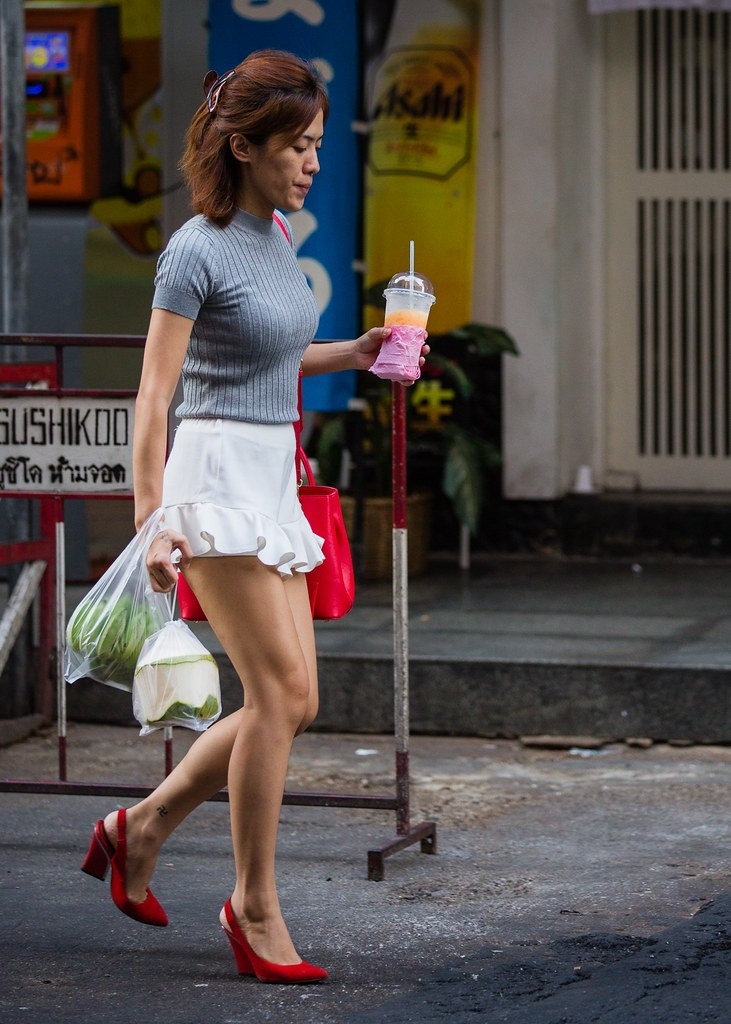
[{"left": 134, "top": 646, "right": 220, "bottom": 725}]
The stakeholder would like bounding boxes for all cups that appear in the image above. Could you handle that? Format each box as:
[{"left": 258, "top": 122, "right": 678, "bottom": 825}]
[{"left": 377, "top": 271, "right": 437, "bottom": 380}]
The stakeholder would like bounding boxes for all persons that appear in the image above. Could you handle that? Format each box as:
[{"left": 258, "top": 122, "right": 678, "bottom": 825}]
[{"left": 81, "top": 50, "right": 431, "bottom": 983}]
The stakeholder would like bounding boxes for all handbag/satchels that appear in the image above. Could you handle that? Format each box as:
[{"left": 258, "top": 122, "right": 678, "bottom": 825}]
[
  {"left": 62, "top": 507, "right": 171, "bottom": 691},
  {"left": 176, "top": 359, "right": 355, "bottom": 619},
  {"left": 132, "top": 553, "right": 223, "bottom": 737}
]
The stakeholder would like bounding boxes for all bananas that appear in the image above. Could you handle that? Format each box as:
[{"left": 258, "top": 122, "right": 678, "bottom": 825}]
[{"left": 65, "top": 591, "right": 157, "bottom": 679}]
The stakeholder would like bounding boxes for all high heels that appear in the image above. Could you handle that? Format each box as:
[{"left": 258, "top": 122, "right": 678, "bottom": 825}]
[
  {"left": 81, "top": 808, "right": 169, "bottom": 927},
  {"left": 219, "top": 894, "right": 329, "bottom": 983}
]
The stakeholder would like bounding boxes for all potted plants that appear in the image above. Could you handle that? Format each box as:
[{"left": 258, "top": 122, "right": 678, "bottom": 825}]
[{"left": 318, "top": 284, "right": 519, "bottom": 582}]
